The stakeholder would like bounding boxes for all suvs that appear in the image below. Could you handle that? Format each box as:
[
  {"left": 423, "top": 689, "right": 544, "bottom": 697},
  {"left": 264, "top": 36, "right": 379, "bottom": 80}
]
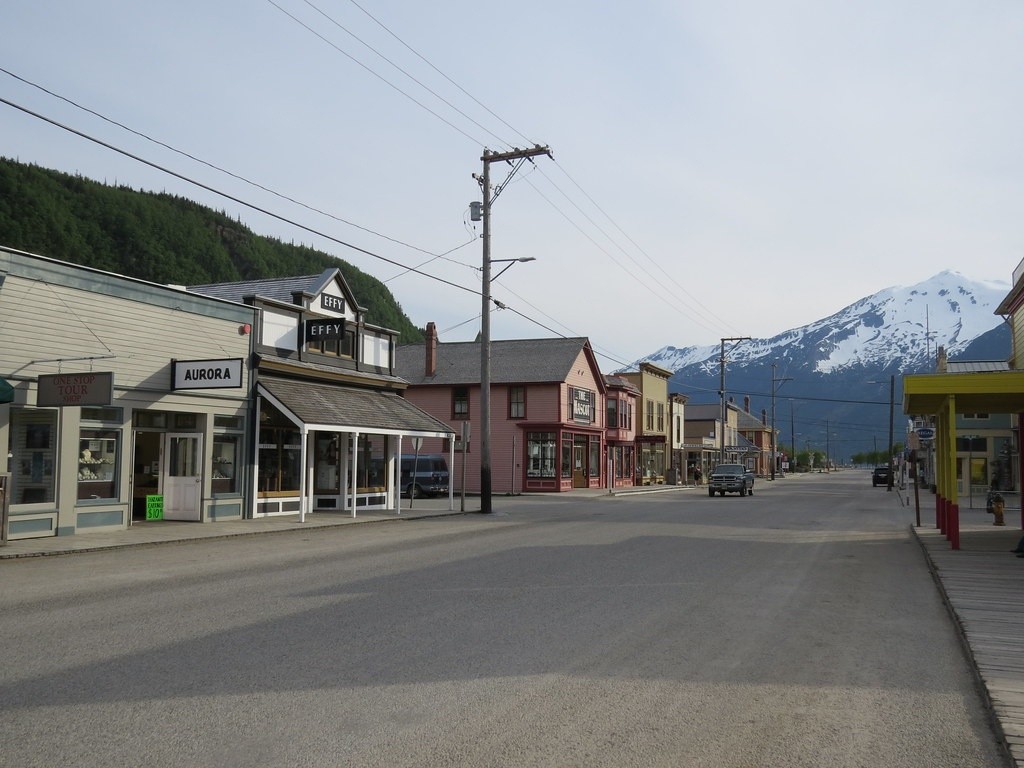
[
  {"left": 871, "top": 467, "right": 895, "bottom": 488},
  {"left": 707, "top": 464, "right": 755, "bottom": 496}
]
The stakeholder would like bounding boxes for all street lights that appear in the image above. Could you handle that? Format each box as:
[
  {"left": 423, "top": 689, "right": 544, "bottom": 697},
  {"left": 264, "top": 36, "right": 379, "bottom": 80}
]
[{"left": 479, "top": 255, "right": 536, "bottom": 513}]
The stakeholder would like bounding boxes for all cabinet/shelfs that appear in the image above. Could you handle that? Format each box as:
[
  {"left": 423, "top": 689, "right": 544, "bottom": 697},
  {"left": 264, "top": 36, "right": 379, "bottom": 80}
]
[
  {"left": 212, "top": 434, "right": 237, "bottom": 493},
  {"left": 76, "top": 430, "right": 118, "bottom": 499}
]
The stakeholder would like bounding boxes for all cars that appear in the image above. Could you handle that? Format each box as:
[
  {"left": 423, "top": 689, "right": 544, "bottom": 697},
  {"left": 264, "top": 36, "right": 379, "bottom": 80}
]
[{"left": 370, "top": 453, "right": 450, "bottom": 499}]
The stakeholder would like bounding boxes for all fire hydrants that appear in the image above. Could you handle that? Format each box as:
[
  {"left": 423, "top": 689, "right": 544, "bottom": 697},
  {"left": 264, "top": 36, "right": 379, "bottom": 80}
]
[{"left": 988, "top": 494, "right": 1007, "bottom": 527}]
